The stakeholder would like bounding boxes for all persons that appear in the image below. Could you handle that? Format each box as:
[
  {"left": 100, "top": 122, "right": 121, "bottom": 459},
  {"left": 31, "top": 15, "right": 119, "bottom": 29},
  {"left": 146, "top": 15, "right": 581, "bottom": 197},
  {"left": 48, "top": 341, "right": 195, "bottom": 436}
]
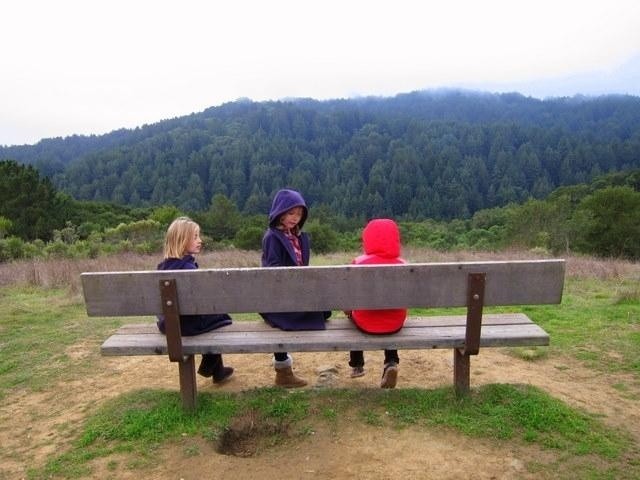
[
  {"left": 343, "top": 218, "right": 409, "bottom": 391},
  {"left": 155, "top": 215, "right": 235, "bottom": 384},
  {"left": 258, "top": 188, "right": 333, "bottom": 387}
]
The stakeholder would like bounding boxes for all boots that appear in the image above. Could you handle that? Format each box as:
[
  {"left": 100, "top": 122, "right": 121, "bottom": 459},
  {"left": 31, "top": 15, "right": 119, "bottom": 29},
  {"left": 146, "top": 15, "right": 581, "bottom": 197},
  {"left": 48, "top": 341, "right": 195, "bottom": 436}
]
[{"left": 272, "top": 354, "right": 309, "bottom": 388}]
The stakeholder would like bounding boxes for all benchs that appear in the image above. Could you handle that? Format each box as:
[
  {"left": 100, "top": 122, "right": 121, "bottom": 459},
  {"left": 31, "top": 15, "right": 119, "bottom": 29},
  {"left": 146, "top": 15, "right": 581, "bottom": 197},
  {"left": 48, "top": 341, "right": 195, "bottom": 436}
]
[{"left": 80, "top": 260, "right": 566, "bottom": 411}]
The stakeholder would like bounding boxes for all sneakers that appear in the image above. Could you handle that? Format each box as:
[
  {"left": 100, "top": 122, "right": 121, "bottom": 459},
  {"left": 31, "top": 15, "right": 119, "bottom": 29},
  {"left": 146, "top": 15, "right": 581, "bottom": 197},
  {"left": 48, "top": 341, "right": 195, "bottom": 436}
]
[
  {"left": 197, "top": 358, "right": 233, "bottom": 384},
  {"left": 380, "top": 361, "right": 398, "bottom": 389},
  {"left": 349, "top": 367, "right": 366, "bottom": 378}
]
